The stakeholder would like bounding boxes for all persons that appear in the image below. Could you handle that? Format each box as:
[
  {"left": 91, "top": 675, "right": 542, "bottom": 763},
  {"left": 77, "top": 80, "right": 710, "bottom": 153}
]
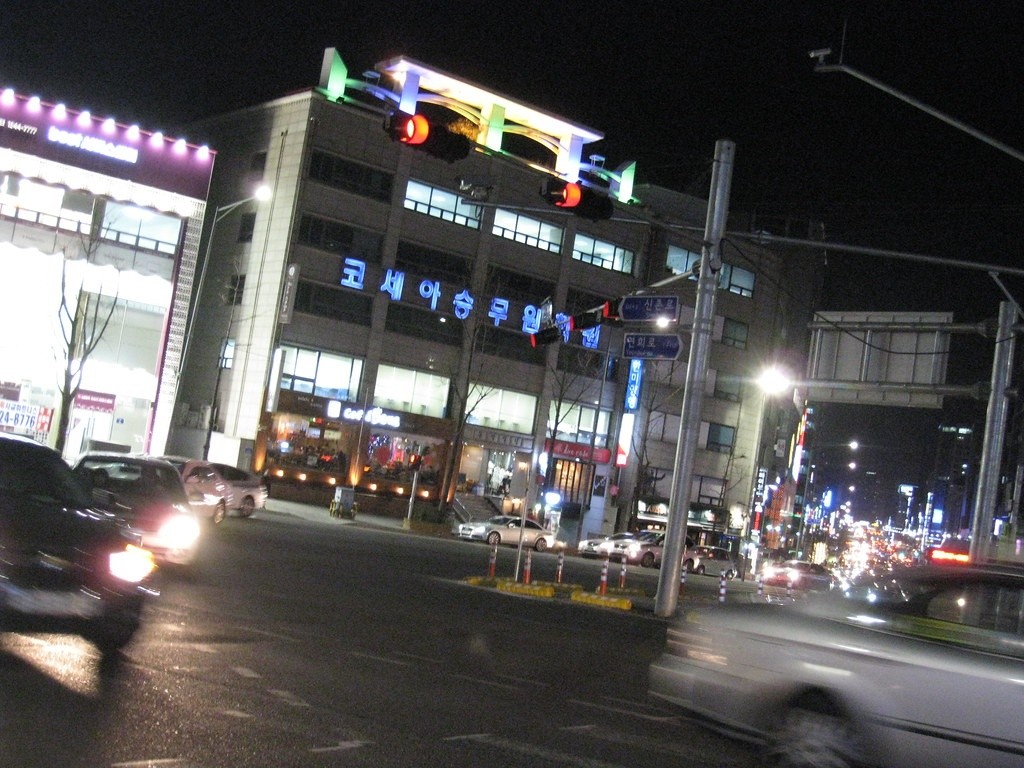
[{"left": 527, "top": 508, "right": 534, "bottom": 519}]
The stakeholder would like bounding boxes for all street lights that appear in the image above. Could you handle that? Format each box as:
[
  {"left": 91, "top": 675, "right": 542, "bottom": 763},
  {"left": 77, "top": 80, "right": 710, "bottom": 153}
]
[
  {"left": 795, "top": 440, "right": 861, "bottom": 558},
  {"left": 573, "top": 317, "right": 671, "bottom": 538},
  {"left": 164, "top": 185, "right": 273, "bottom": 455},
  {"left": 741, "top": 366, "right": 792, "bottom": 583}
]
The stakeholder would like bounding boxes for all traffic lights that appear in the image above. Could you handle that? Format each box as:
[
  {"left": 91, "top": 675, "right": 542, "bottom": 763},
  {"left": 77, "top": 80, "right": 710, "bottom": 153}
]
[
  {"left": 566, "top": 311, "right": 602, "bottom": 331},
  {"left": 407, "top": 453, "right": 422, "bottom": 471},
  {"left": 538, "top": 178, "right": 614, "bottom": 221},
  {"left": 529, "top": 325, "right": 560, "bottom": 349},
  {"left": 382, "top": 109, "right": 471, "bottom": 164},
  {"left": 603, "top": 296, "right": 625, "bottom": 320}
]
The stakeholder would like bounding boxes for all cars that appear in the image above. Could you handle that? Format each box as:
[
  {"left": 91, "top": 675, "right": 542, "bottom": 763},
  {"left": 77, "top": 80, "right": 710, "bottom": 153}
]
[
  {"left": 73, "top": 450, "right": 202, "bottom": 568},
  {"left": 458, "top": 514, "right": 557, "bottom": 552},
  {"left": 607, "top": 529, "right": 701, "bottom": 574},
  {"left": 156, "top": 454, "right": 235, "bottom": 527},
  {"left": 206, "top": 460, "right": 269, "bottom": 519},
  {"left": 763, "top": 559, "right": 835, "bottom": 593},
  {"left": 0, "top": 430, "right": 152, "bottom": 652},
  {"left": 694, "top": 544, "right": 738, "bottom": 581},
  {"left": 577, "top": 532, "right": 634, "bottom": 560},
  {"left": 644, "top": 561, "right": 1023, "bottom": 768}
]
